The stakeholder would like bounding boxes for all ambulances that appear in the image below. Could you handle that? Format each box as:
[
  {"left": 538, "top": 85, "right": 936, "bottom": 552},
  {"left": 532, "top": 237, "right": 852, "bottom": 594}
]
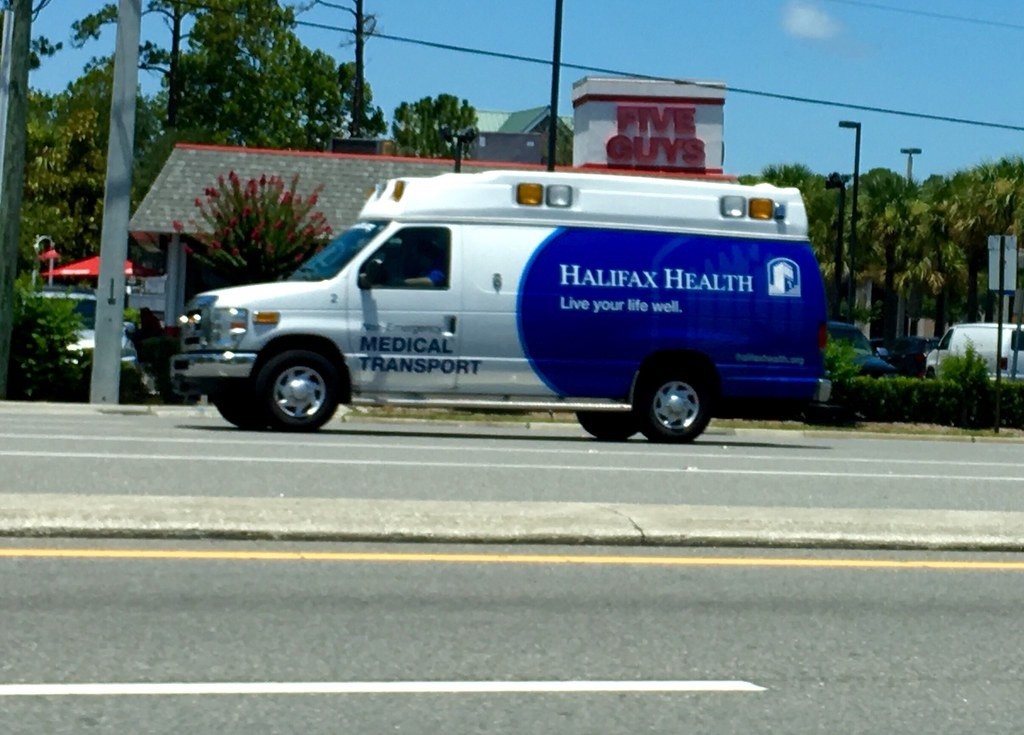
[{"left": 172, "top": 170, "right": 834, "bottom": 446}]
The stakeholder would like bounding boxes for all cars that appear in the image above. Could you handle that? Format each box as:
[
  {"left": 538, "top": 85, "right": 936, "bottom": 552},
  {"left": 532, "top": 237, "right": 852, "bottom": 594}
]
[
  {"left": 870, "top": 337, "right": 938, "bottom": 382},
  {"left": 32, "top": 287, "right": 142, "bottom": 372},
  {"left": 826, "top": 322, "right": 901, "bottom": 381}
]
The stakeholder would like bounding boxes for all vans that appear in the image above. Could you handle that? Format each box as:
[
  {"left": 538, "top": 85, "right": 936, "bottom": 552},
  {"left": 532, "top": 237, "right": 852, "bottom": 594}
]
[{"left": 924, "top": 323, "right": 1023, "bottom": 382}]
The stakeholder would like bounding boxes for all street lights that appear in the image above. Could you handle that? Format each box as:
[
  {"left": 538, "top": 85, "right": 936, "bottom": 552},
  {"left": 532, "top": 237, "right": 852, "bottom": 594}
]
[
  {"left": 826, "top": 172, "right": 845, "bottom": 322},
  {"left": 838, "top": 120, "right": 862, "bottom": 323},
  {"left": 902, "top": 148, "right": 923, "bottom": 185}
]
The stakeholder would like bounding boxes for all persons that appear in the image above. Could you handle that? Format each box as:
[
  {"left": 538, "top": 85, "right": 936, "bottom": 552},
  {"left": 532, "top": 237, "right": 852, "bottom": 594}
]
[{"left": 403, "top": 241, "right": 447, "bottom": 287}]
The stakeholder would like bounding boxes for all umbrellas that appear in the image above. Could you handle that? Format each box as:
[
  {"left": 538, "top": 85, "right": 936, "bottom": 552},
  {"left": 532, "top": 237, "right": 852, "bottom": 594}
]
[
  {"left": 38, "top": 255, "right": 165, "bottom": 277},
  {"left": 39, "top": 247, "right": 63, "bottom": 260}
]
